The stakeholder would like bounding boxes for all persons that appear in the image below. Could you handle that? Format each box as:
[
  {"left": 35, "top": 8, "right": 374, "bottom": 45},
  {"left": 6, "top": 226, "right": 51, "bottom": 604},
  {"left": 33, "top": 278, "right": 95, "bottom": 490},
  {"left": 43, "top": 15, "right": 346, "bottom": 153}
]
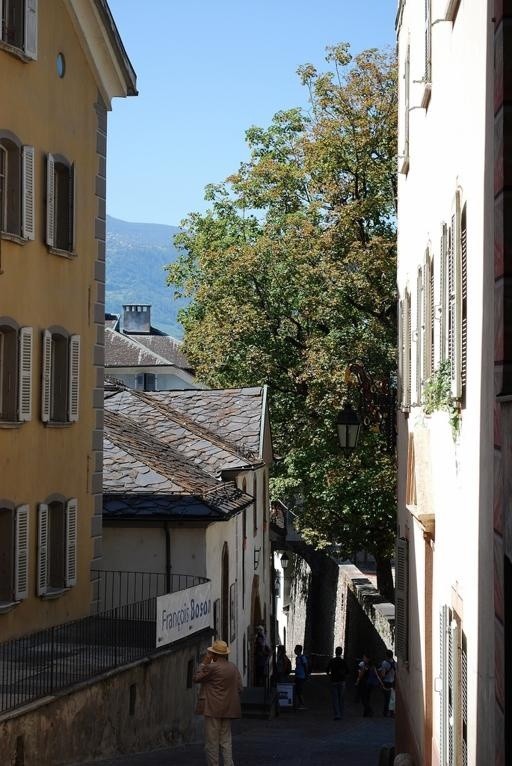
[
  {"left": 253, "top": 632, "right": 273, "bottom": 686},
  {"left": 354, "top": 651, "right": 374, "bottom": 718},
  {"left": 276, "top": 645, "right": 291, "bottom": 678},
  {"left": 192, "top": 640, "right": 244, "bottom": 766},
  {"left": 376, "top": 650, "right": 397, "bottom": 717},
  {"left": 294, "top": 644, "right": 308, "bottom": 708},
  {"left": 327, "top": 647, "right": 349, "bottom": 720}
]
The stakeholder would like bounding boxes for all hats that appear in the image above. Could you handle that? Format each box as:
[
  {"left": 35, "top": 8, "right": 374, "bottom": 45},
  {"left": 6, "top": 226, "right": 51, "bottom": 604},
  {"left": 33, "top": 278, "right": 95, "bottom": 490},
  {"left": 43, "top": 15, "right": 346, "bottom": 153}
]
[{"left": 207, "top": 640, "right": 232, "bottom": 655}]
